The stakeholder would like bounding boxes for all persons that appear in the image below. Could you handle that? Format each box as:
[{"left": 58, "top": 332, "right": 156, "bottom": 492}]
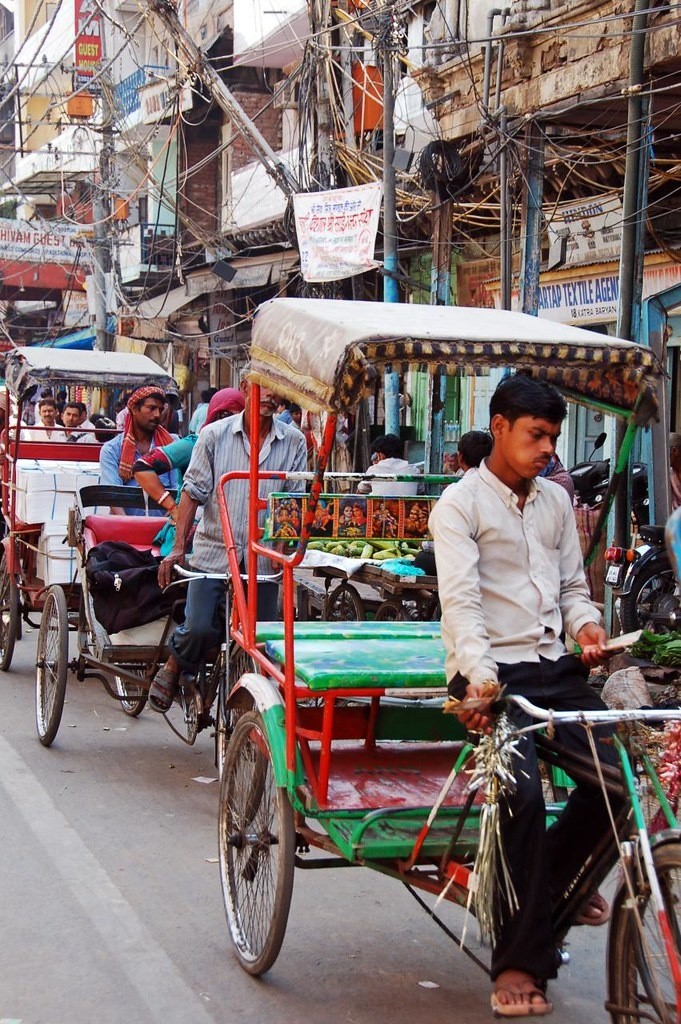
[
  {"left": 538, "top": 452, "right": 574, "bottom": 505},
  {"left": 442, "top": 452, "right": 452, "bottom": 475},
  {"left": 354, "top": 434, "right": 425, "bottom": 495},
  {"left": 448, "top": 453, "right": 465, "bottom": 476},
  {"left": 187, "top": 387, "right": 219, "bottom": 434},
  {"left": 458, "top": 431, "right": 494, "bottom": 471},
  {"left": 131, "top": 388, "right": 246, "bottom": 623},
  {"left": 147, "top": 363, "right": 309, "bottom": 712},
  {"left": 98, "top": 384, "right": 180, "bottom": 518},
  {"left": 663, "top": 432, "right": 681, "bottom": 592},
  {"left": 274, "top": 398, "right": 349, "bottom": 458},
  {"left": 24, "top": 388, "right": 133, "bottom": 444},
  {"left": 425, "top": 374, "right": 638, "bottom": 1018}
]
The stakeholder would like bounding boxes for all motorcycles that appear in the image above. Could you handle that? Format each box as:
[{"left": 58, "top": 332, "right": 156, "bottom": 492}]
[{"left": 563, "top": 431, "right": 680, "bottom": 659}]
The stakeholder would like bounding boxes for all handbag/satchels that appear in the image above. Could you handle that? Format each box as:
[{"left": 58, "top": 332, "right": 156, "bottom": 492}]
[{"left": 85, "top": 541, "right": 178, "bottom": 635}]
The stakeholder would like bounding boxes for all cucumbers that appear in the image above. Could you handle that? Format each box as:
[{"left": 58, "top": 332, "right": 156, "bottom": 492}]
[{"left": 305, "top": 540, "right": 420, "bottom": 559}]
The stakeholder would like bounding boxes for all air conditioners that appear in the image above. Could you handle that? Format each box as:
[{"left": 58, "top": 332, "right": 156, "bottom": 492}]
[
  {"left": 273, "top": 79, "right": 297, "bottom": 109},
  {"left": 291, "top": 80, "right": 303, "bottom": 107}
]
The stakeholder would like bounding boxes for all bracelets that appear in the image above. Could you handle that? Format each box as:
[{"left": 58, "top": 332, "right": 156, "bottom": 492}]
[
  {"left": 167, "top": 503, "right": 176, "bottom": 512},
  {"left": 158, "top": 491, "right": 170, "bottom": 504}
]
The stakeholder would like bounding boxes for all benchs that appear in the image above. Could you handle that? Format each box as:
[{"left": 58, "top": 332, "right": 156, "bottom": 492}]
[
  {"left": 82, "top": 514, "right": 169, "bottom": 571},
  {"left": 265, "top": 639, "right": 448, "bottom": 810},
  {"left": 253, "top": 620, "right": 442, "bottom": 641}
]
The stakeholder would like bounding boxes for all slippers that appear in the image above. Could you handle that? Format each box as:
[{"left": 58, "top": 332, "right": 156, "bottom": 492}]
[
  {"left": 491, "top": 975, "right": 550, "bottom": 1016},
  {"left": 148, "top": 668, "right": 179, "bottom": 712},
  {"left": 575, "top": 893, "right": 610, "bottom": 925}
]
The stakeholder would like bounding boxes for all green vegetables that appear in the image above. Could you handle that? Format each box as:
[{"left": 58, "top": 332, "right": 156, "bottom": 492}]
[{"left": 630, "top": 628, "right": 681, "bottom": 668}]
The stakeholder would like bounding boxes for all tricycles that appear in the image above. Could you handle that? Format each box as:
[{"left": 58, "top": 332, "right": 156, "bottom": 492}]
[
  {"left": 1, "top": 345, "right": 286, "bottom": 751},
  {"left": 212, "top": 296, "right": 681, "bottom": 1024}
]
